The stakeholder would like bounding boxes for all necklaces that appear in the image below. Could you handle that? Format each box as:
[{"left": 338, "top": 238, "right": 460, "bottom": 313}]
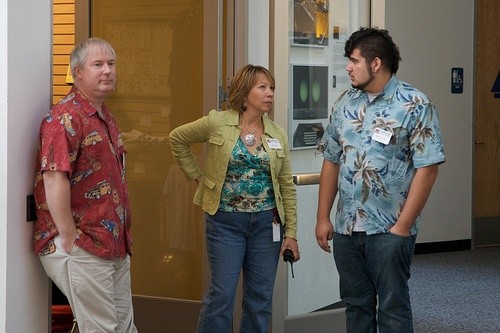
[{"left": 239, "top": 117, "right": 262, "bottom": 146}]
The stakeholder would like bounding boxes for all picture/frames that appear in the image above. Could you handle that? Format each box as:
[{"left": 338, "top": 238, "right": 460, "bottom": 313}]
[
  {"left": 288, "top": 0, "right": 330, "bottom": 48},
  {"left": 287, "top": 61, "right": 332, "bottom": 152}
]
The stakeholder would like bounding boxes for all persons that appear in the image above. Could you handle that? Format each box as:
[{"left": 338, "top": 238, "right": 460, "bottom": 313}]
[
  {"left": 33, "top": 37, "right": 139, "bottom": 333},
  {"left": 169, "top": 65, "right": 301, "bottom": 333},
  {"left": 315, "top": 26, "right": 446, "bottom": 333}
]
